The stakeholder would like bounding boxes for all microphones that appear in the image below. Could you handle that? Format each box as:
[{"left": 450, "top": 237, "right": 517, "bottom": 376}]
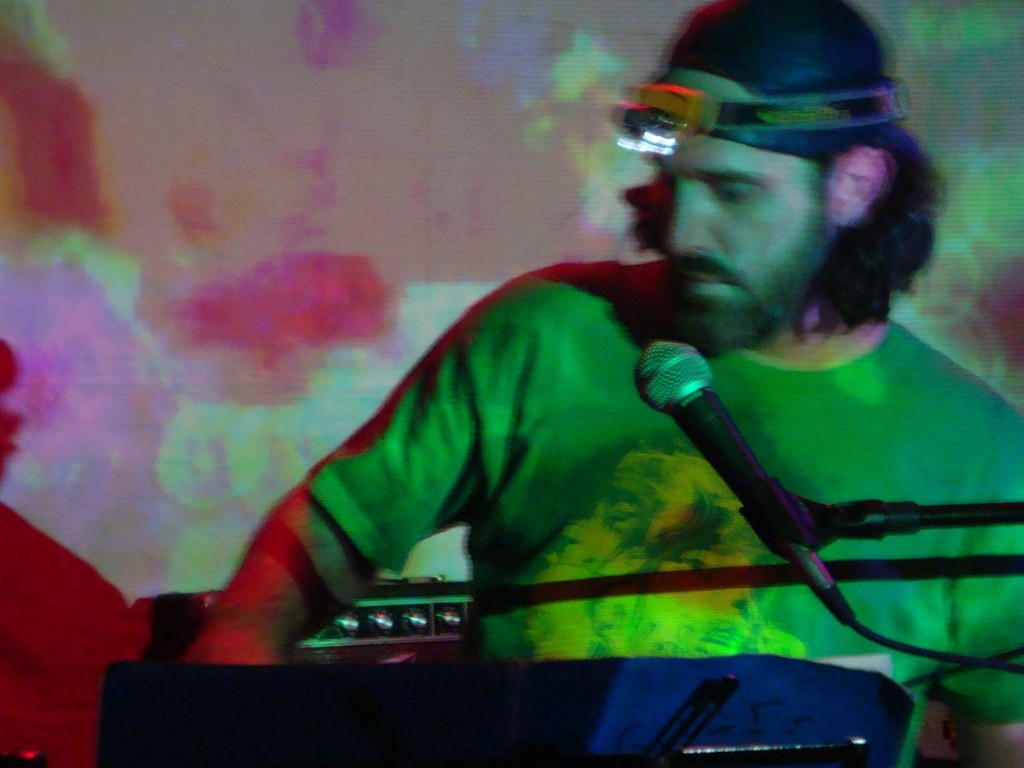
[{"left": 633, "top": 340, "right": 855, "bottom": 626}]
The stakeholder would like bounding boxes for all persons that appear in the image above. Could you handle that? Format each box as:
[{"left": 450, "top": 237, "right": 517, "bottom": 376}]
[{"left": 181, "top": 0, "right": 1024, "bottom": 768}]
